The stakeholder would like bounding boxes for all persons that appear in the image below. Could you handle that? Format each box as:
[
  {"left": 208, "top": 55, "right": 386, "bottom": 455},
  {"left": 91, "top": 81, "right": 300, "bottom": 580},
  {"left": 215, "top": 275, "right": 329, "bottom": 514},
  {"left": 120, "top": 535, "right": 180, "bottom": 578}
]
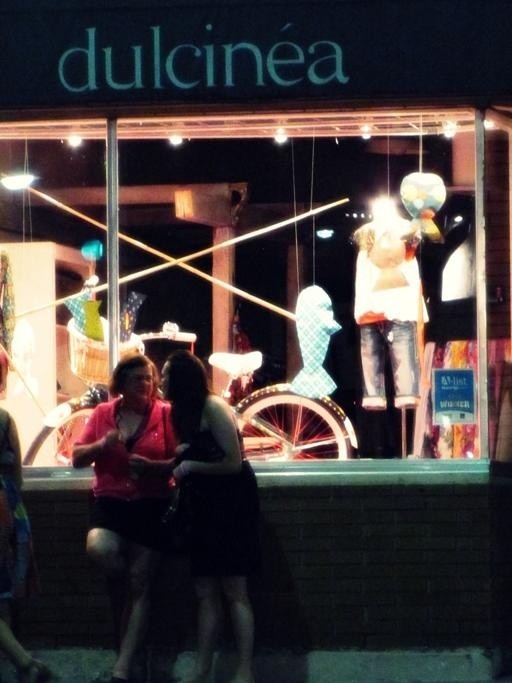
[
  {"left": 1, "top": 342, "right": 55, "bottom": 682},
  {"left": 21, "top": 347, "right": 260, "bottom": 683},
  {"left": 351, "top": 190, "right": 422, "bottom": 409}
]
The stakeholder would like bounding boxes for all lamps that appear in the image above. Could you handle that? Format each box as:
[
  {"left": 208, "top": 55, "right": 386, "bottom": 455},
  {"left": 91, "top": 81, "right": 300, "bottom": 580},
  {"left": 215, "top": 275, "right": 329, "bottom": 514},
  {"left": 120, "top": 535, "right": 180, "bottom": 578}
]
[{"left": 397, "top": 112, "right": 447, "bottom": 219}]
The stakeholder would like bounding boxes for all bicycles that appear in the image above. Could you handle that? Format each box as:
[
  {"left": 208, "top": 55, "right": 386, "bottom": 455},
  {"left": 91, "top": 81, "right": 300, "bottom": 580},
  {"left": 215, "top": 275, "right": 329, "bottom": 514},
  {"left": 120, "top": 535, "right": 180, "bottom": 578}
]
[{"left": 23, "top": 274, "right": 354, "bottom": 466}]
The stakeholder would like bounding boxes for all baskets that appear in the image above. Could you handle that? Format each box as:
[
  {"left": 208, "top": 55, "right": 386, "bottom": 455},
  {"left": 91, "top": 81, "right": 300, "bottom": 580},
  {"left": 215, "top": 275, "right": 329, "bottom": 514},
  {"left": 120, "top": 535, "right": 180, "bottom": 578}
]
[{"left": 66, "top": 316, "right": 145, "bottom": 386}]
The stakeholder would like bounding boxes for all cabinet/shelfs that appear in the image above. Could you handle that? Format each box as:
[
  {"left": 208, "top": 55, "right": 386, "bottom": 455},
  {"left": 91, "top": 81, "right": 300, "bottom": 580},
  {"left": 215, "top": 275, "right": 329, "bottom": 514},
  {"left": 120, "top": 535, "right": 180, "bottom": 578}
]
[{"left": 1, "top": 240, "right": 99, "bottom": 468}]
